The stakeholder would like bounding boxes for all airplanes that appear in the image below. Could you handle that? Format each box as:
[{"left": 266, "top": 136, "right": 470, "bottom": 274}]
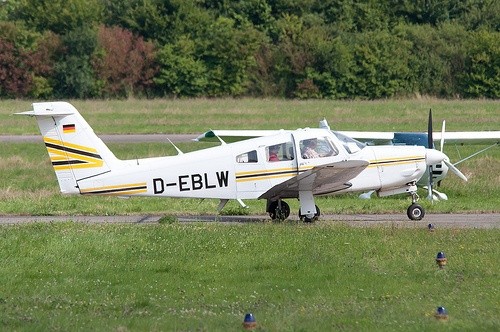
[{"left": 14, "top": 101, "right": 499, "bottom": 222}]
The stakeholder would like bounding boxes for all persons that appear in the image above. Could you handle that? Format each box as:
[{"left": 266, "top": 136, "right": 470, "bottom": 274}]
[
  {"left": 269, "top": 144, "right": 283, "bottom": 161},
  {"left": 302, "top": 137, "right": 336, "bottom": 159}
]
